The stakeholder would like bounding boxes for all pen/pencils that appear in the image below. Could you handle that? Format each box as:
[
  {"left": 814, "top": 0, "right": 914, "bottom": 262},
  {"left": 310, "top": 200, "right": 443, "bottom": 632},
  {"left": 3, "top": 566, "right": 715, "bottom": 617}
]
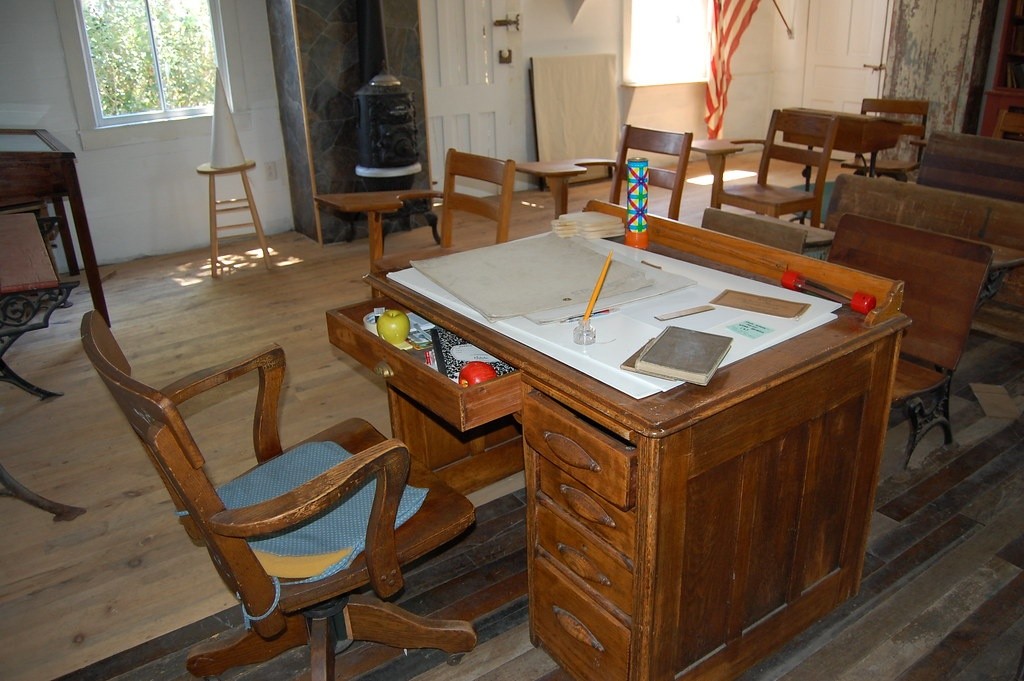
[
  {"left": 581, "top": 249, "right": 614, "bottom": 328},
  {"left": 641, "top": 260, "right": 662, "bottom": 269}
]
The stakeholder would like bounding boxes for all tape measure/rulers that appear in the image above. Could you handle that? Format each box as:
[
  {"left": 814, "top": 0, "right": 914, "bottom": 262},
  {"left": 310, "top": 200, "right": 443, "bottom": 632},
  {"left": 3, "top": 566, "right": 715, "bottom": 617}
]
[{"left": 654, "top": 305, "right": 715, "bottom": 321}]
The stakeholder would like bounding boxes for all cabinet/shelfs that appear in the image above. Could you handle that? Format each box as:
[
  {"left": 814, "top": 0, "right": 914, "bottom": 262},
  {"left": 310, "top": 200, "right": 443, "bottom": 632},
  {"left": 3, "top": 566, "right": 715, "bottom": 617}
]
[
  {"left": 978, "top": 0, "right": 1024, "bottom": 142},
  {"left": 325, "top": 199, "right": 915, "bottom": 681}
]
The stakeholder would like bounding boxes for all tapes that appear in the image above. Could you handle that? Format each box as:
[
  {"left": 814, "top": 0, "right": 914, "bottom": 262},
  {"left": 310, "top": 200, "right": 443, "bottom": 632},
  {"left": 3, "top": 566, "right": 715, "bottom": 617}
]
[{"left": 363, "top": 309, "right": 391, "bottom": 337}]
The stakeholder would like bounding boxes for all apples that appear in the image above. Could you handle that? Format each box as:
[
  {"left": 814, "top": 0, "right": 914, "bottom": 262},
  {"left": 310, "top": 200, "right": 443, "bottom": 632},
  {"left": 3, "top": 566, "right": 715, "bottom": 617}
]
[
  {"left": 459, "top": 363, "right": 497, "bottom": 388},
  {"left": 377, "top": 309, "right": 409, "bottom": 345}
]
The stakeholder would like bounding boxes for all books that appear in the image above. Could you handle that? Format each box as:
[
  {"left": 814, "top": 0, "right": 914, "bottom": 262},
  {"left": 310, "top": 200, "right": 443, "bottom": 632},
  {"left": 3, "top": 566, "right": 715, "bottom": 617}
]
[{"left": 620, "top": 325, "right": 734, "bottom": 385}]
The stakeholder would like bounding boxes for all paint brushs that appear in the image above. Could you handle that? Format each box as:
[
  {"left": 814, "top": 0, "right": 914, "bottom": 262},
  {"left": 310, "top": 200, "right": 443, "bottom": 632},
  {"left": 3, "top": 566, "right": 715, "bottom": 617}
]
[{"left": 560, "top": 308, "right": 620, "bottom": 323}]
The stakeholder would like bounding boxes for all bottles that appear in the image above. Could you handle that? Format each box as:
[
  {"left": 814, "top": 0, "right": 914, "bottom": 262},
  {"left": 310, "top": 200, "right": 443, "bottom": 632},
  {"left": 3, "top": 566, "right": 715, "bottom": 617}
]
[{"left": 573, "top": 318, "right": 596, "bottom": 345}]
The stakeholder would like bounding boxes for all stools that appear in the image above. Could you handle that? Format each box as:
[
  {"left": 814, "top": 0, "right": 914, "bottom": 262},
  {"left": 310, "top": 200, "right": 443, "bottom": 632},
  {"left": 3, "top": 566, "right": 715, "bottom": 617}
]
[{"left": 196, "top": 160, "right": 273, "bottom": 276}]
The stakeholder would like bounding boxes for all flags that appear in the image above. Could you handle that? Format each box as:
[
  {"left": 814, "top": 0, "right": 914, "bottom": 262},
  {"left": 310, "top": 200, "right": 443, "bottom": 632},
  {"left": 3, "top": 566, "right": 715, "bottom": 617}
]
[{"left": 704, "top": 0, "right": 759, "bottom": 140}]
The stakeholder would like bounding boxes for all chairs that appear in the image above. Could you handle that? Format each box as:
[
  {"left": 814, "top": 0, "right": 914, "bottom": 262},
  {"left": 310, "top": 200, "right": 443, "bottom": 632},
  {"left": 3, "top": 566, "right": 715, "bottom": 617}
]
[
  {"left": 700, "top": 100, "right": 1024, "bottom": 472},
  {"left": 512, "top": 125, "right": 693, "bottom": 222},
  {"left": 686, "top": 107, "right": 839, "bottom": 225},
  {"left": 312, "top": 147, "right": 515, "bottom": 300},
  {"left": 78, "top": 306, "right": 477, "bottom": 681}
]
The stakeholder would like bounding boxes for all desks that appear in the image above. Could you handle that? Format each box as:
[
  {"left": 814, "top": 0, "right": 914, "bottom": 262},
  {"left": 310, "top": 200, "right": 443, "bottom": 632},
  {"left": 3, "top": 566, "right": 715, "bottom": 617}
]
[
  {"left": 0, "top": 212, "right": 88, "bottom": 522},
  {"left": 781, "top": 107, "right": 902, "bottom": 225},
  {"left": 742, "top": 214, "right": 835, "bottom": 262},
  {"left": 0, "top": 127, "right": 111, "bottom": 328}
]
